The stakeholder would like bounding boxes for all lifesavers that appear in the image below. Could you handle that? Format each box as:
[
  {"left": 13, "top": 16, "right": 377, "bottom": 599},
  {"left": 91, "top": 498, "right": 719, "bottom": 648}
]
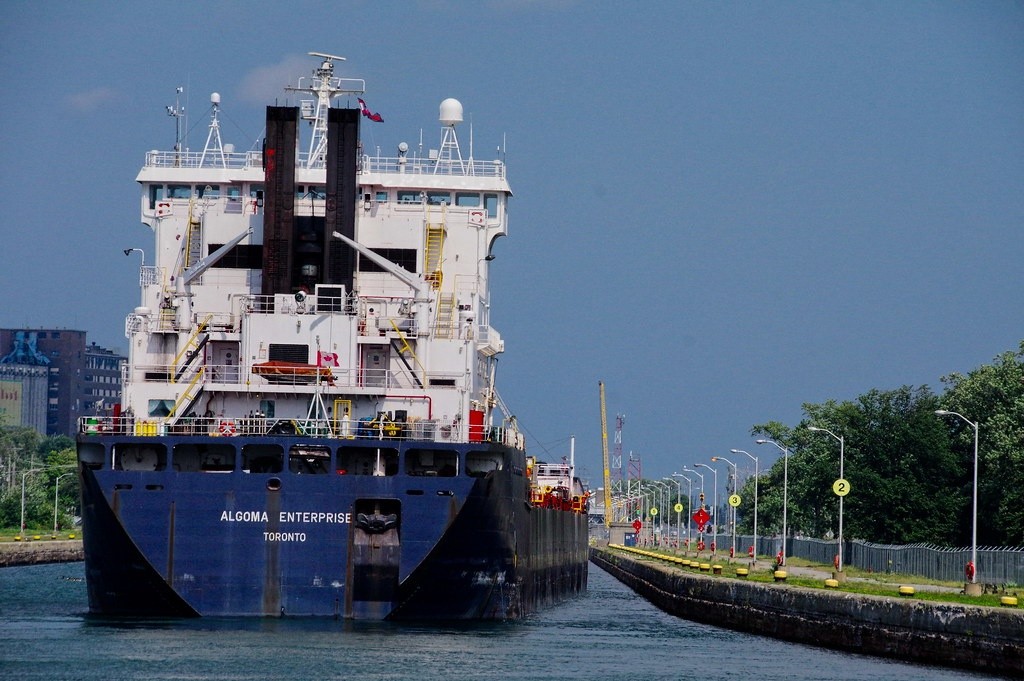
[
  {"left": 633, "top": 535, "right": 785, "bottom": 566},
  {"left": 218, "top": 420, "right": 237, "bottom": 438},
  {"left": 964, "top": 562, "right": 975, "bottom": 579},
  {"left": 833, "top": 554, "right": 840, "bottom": 569}
]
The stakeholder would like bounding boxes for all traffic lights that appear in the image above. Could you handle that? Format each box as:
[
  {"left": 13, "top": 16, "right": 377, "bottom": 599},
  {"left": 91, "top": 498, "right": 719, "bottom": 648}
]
[{"left": 699, "top": 494, "right": 705, "bottom": 502}]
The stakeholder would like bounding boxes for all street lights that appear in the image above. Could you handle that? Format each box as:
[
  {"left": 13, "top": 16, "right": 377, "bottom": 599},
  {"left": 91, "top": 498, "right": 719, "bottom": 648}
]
[
  {"left": 610, "top": 473, "right": 691, "bottom": 558},
  {"left": 712, "top": 456, "right": 741, "bottom": 566},
  {"left": 757, "top": 440, "right": 789, "bottom": 578},
  {"left": 808, "top": 427, "right": 851, "bottom": 583},
  {"left": 20, "top": 468, "right": 40, "bottom": 539},
  {"left": 694, "top": 463, "right": 717, "bottom": 562},
  {"left": 54, "top": 473, "right": 72, "bottom": 533},
  {"left": 683, "top": 468, "right": 710, "bottom": 559},
  {"left": 935, "top": 411, "right": 982, "bottom": 596},
  {"left": 730, "top": 449, "right": 758, "bottom": 573}
]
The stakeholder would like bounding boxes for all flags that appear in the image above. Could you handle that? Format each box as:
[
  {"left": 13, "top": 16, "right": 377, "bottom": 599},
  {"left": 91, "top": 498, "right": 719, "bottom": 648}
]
[
  {"left": 358, "top": 98, "right": 384, "bottom": 122},
  {"left": 317, "top": 352, "right": 340, "bottom": 366}
]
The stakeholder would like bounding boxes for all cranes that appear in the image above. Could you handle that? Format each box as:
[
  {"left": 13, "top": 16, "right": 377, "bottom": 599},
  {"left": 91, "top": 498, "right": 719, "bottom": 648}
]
[{"left": 598, "top": 382, "right": 613, "bottom": 538}]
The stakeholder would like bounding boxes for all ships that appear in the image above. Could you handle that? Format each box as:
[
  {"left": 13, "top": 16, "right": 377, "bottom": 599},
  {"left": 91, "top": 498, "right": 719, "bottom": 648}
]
[{"left": 76, "top": 50, "right": 589, "bottom": 624}]
